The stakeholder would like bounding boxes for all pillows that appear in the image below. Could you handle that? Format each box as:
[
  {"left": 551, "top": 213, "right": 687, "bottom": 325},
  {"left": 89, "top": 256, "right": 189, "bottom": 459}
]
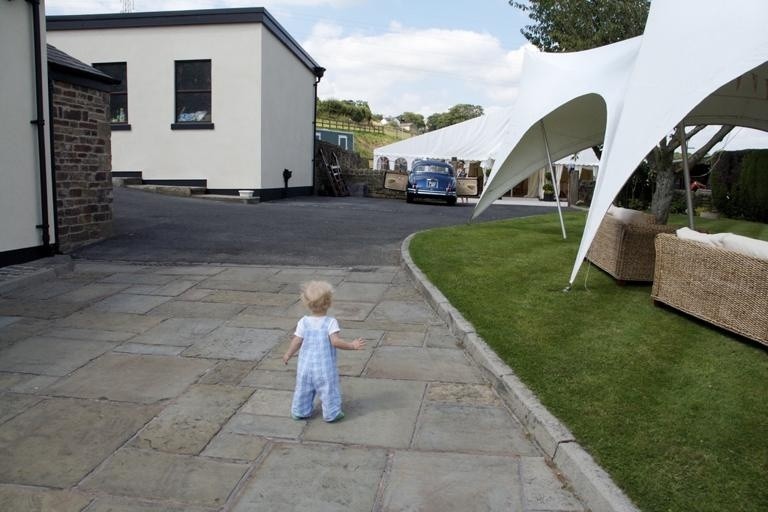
[
  {"left": 725, "top": 233, "right": 768, "bottom": 262},
  {"left": 614, "top": 208, "right": 647, "bottom": 225},
  {"left": 607, "top": 205, "right": 618, "bottom": 215},
  {"left": 676, "top": 227, "right": 731, "bottom": 250}
]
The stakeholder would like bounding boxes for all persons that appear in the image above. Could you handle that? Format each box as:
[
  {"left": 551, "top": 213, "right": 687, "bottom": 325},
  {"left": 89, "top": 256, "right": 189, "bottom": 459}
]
[
  {"left": 282, "top": 279, "right": 368, "bottom": 423},
  {"left": 456, "top": 162, "right": 468, "bottom": 203}
]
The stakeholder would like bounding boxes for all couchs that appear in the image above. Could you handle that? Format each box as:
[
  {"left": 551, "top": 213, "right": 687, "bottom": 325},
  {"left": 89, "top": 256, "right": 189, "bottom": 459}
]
[
  {"left": 585, "top": 204, "right": 676, "bottom": 283},
  {"left": 650, "top": 227, "right": 768, "bottom": 347}
]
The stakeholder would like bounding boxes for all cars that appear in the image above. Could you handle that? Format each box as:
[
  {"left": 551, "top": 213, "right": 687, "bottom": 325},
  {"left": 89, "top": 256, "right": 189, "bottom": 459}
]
[{"left": 407, "top": 159, "right": 458, "bottom": 205}]
